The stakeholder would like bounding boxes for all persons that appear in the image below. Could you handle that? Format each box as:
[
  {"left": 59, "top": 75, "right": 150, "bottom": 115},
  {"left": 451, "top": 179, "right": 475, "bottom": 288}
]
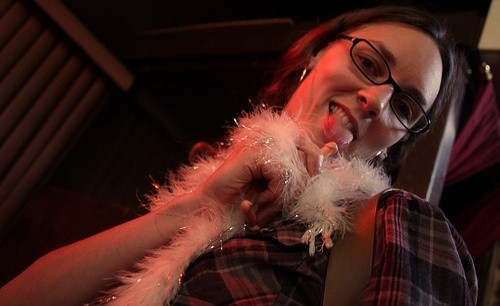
[{"left": 1, "top": 7, "right": 487, "bottom": 305}]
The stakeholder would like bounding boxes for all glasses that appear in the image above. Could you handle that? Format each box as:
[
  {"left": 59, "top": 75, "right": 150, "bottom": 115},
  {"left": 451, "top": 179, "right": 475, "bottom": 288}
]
[{"left": 331, "top": 33, "right": 433, "bottom": 145}]
